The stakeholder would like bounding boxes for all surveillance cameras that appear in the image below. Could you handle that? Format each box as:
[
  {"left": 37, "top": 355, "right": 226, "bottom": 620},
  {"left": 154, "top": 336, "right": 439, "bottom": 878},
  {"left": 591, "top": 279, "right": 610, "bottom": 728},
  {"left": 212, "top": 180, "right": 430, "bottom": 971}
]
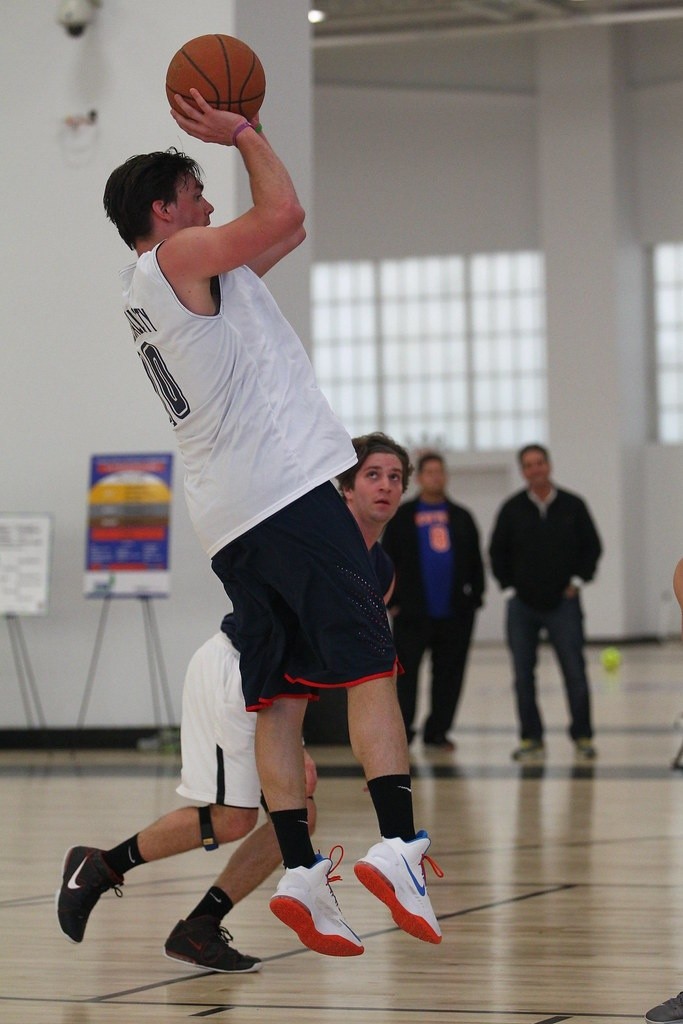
[{"left": 56, "top": 0, "right": 97, "bottom": 36}]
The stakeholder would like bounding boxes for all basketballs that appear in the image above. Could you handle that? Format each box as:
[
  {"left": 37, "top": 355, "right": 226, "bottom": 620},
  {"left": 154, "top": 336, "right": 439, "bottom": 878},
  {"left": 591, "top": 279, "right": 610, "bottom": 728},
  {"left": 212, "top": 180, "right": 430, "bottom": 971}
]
[{"left": 166, "top": 34, "right": 266, "bottom": 129}]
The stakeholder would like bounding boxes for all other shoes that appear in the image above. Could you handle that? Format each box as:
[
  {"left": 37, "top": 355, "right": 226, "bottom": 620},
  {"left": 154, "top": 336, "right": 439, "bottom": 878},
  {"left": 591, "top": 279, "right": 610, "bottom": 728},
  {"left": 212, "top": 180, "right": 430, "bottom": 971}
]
[
  {"left": 511, "top": 740, "right": 551, "bottom": 764},
  {"left": 570, "top": 736, "right": 602, "bottom": 766}
]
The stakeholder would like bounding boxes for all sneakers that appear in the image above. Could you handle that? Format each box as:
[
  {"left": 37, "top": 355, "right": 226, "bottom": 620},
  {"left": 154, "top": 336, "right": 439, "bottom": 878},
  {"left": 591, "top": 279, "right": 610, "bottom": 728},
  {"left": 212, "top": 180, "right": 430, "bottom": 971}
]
[
  {"left": 355, "top": 837, "right": 448, "bottom": 945},
  {"left": 54, "top": 844, "right": 119, "bottom": 945},
  {"left": 164, "top": 913, "right": 265, "bottom": 972},
  {"left": 269, "top": 856, "right": 368, "bottom": 959}
]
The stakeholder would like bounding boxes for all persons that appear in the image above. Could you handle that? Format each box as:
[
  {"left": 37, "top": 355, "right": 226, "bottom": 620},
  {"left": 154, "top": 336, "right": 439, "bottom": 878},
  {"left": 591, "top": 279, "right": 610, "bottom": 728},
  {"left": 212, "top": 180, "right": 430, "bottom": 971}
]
[
  {"left": 57, "top": 431, "right": 418, "bottom": 974},
  {"left": 487, "top": 441, "right": 604, "bottom": 763},
  {"left": 382, "top": 447, "right": 486, "bottom": 752},
  {"left": 104, "top": 86, "right": 451, "bottom": 958}
]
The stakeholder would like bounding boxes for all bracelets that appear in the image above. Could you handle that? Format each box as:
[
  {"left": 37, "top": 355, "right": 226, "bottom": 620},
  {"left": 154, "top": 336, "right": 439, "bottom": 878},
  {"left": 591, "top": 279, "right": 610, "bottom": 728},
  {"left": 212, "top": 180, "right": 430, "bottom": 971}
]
[
  {"left": 255, "top": 123, "right": 263, "bottom": 135},
  {"left": 230, "top": 120, "right": 253, "bottom": 148}
]
